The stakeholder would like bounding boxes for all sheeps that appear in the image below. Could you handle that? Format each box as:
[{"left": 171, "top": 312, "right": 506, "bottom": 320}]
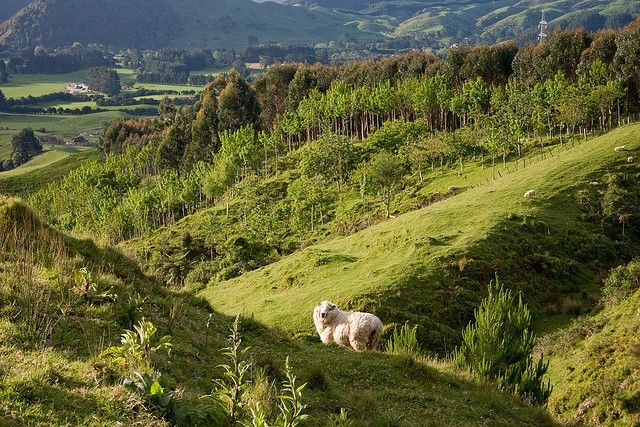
[
  {"left": 524, "top": 190, "right": 535, "bottom": 198},
  {"left": 313, "top": 300, "right": 383, "bottom": 352},
  {"left": 628, "top": 157, "right": 633, "bottom": 163},
  {"left": 614, "top": 146, "right": 624, "bottom": 151},
  {"left": 590, "top": 182, "right": 598, "bottom": 185}
]
